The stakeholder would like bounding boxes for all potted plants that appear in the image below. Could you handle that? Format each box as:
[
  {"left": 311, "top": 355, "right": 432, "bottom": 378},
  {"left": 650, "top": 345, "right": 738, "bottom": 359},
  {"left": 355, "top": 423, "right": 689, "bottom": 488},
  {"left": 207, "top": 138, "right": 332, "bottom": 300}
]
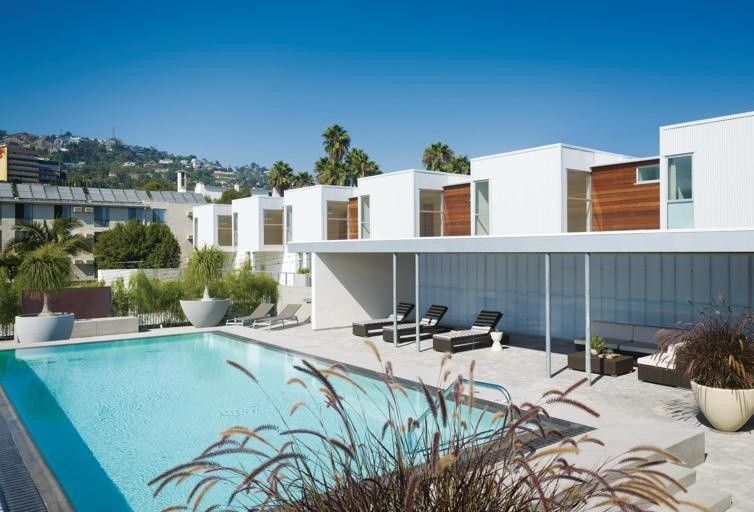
[
  {"left": 11, "top": 241, "right": 74, "bottom": 344},
  {"left": 650, "top": 286, "right": 754, "bottom": 432},
  {"left": 178, "top": 242, "right": 230, "bottom": 328}
]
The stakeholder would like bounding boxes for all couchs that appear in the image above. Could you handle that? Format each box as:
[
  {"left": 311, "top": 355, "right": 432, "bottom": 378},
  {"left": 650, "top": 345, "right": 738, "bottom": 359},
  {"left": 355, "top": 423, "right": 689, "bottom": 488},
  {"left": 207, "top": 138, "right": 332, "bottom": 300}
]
[{"left": 573, "top": 320, "right": 693, "bottom": 388}]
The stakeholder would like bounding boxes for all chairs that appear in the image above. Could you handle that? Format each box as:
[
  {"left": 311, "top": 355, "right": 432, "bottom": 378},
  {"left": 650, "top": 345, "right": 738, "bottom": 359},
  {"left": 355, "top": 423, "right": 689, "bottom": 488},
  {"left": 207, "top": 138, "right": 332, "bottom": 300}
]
[
  {"left": 351, "top": 302, "right": 503, "bottom": 355},
  {"left": 224, "top": 302, "right": 301, "bottom": 331}
]
